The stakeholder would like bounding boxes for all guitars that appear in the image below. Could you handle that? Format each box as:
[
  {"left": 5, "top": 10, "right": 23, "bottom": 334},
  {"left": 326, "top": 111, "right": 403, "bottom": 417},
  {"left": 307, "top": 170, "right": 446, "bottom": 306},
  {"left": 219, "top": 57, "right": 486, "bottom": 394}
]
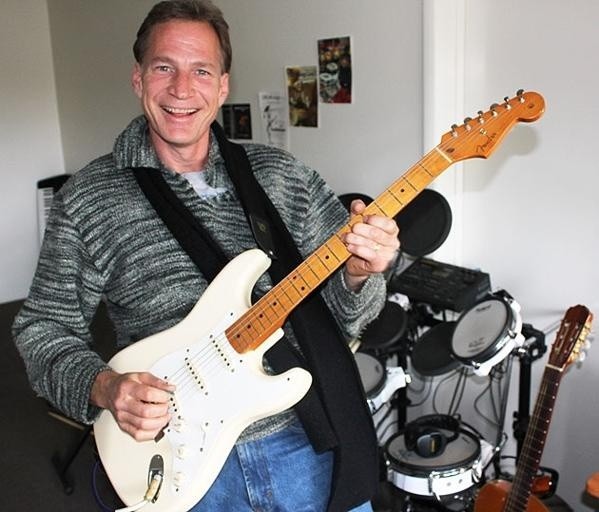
[
  {"left": 93, "top": 89, "right": 545, "bottom": 511},
  {"left": 472, "top": 305, "right": 594, "bottom": 511}
]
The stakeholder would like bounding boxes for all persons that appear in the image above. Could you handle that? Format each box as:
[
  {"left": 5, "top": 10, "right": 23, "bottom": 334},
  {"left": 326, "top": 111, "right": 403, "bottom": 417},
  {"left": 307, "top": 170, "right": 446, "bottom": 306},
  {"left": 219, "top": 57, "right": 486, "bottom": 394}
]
[{"left": 10, "top": 1, "right": 402, "bottom": 512}]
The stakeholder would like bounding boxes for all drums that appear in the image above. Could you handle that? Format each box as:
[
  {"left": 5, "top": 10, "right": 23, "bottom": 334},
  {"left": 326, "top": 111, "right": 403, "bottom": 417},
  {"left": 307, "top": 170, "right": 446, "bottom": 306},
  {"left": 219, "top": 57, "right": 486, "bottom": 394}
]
[
  {"left": 449, "top": 296, "right": 518, "bottom": 364},
  {"left": 350, "top": 350, "right": 387, "bottom": 400},
  {"left": 338, "top": 193, "right": 374, "bottom": 215},
  {"left": 411, "top": 322, "right": 460, "bottom": 376},
  {"left": 381, "top": 423, "right": 485, "bottom": 506},
  {"left": 392, "top": 188, "right": 452, "bottom": 257},
  {"left": 356, "top": 301, "right": 408, "bottom": 351}
]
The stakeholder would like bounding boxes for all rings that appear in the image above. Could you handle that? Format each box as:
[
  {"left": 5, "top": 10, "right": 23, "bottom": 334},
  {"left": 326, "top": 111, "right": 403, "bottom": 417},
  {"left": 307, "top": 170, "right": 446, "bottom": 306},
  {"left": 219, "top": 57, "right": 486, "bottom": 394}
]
[{"left": 373, "top": 243, "right": 381, "bottom": 251}]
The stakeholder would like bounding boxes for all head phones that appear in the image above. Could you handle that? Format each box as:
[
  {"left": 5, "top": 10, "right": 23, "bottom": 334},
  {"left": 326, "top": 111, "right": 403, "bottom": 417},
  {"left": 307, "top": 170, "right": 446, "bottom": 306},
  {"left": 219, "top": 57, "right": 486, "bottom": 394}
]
[{"left": 404, "top": 413, "right": 461, "bottom": 459}]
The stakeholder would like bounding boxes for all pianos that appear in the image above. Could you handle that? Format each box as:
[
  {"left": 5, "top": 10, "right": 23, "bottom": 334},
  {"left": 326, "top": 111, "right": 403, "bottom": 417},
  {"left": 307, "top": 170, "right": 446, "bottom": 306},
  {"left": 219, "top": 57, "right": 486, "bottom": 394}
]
[{"left": 38, "top": 175, "right": 72, "bottom": 246}]
